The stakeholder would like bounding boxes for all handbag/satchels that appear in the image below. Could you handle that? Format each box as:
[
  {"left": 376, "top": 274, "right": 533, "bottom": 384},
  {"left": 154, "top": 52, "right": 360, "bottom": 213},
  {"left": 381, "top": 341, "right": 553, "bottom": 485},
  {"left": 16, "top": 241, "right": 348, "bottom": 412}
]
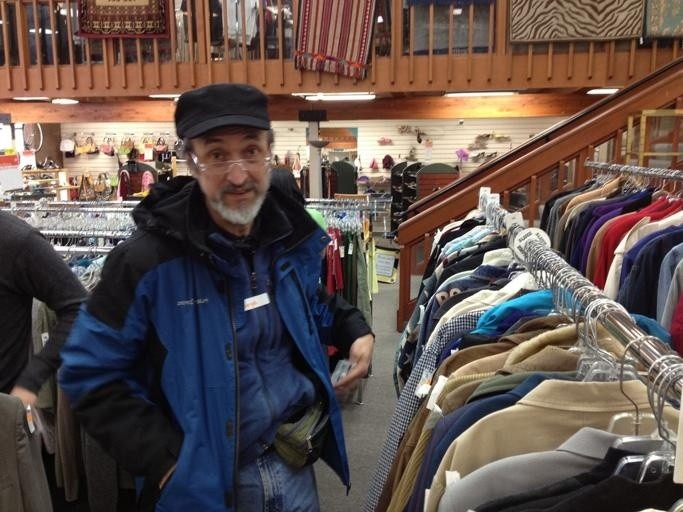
[
  {"left": 59, "top": 133, "right": 169, "bottom": 158},
  {"left": 79, "top": 172, "right": 119, "bottom": 200},
  {"left": 275, "top": 399, "right": 330, "bottom": 470}
]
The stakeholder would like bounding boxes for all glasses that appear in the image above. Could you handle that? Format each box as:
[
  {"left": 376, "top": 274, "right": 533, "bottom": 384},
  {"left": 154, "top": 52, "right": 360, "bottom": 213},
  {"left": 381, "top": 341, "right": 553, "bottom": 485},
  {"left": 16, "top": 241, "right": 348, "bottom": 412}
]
[{"left": 188, "top": 144, "right": 272, "bottom": 175}]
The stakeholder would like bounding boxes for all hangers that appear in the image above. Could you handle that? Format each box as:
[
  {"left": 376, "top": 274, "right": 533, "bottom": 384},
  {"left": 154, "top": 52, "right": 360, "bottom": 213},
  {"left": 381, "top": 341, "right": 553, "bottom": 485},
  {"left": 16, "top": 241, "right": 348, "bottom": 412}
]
[
  {"left": 303, "top": 200, "right": 368, "bottom": 243},
  {"left": 476, "top": 196, "right": 580, "bottom": 277},
  {"left": 538, "top": 275, "right": 682, "bottom": 512},
  {"left": 582, "top": 156, "right": 683, "bottom": 204},
  {"left": 0, "top": 201, "right": 140, "bottom": 292}
]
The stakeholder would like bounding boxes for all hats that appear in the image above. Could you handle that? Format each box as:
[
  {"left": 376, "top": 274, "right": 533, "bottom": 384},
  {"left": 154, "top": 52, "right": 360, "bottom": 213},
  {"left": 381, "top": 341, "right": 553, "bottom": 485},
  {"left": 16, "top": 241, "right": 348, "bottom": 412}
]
[{"left": 174, "top": 83, "right": 270, "bottom": 140}]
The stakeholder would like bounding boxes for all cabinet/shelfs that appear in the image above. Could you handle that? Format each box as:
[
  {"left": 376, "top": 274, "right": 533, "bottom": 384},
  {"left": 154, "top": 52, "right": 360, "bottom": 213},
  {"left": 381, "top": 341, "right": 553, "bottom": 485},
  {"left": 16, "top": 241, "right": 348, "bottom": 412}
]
[
  {"left": 389, "top": 161, "right": 459, "bottom": 239},
  {"left": 22, "top": 168, "right": 82, "bottom": 201}
]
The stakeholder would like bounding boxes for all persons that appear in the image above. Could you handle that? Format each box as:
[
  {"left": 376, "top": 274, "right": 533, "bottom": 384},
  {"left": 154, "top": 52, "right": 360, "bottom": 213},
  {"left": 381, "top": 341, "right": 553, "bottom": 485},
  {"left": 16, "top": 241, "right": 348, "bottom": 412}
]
[
  {"left": 0, "top": 202, "right": 90, "bottom": 511},
  {"left": 51, "top": 82, "right": 376, "bottom": 511}
]
